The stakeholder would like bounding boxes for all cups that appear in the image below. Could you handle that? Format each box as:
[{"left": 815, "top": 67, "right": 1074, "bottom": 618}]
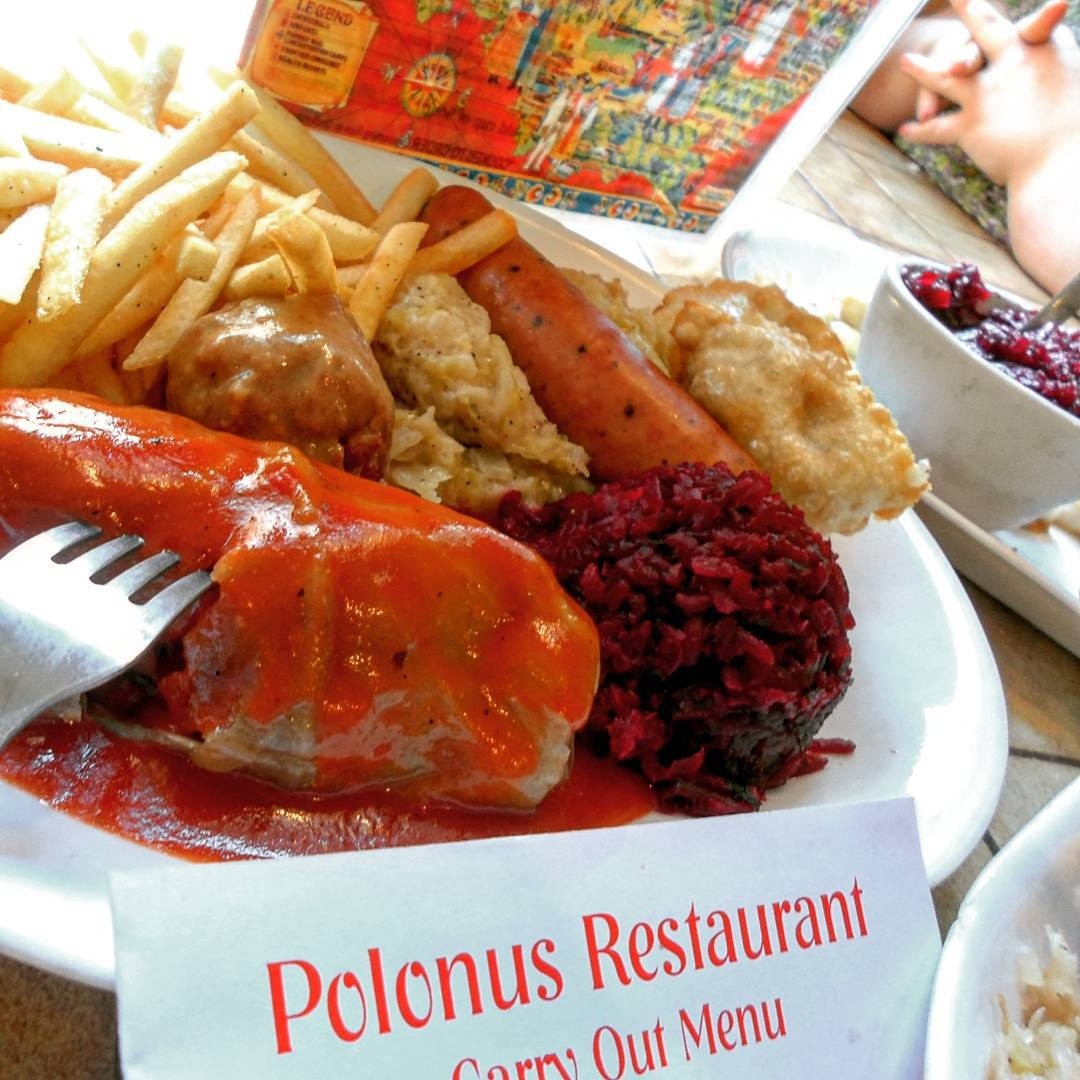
[{"left": 859, "top": 257, "right": 1080, "bottom": 532}]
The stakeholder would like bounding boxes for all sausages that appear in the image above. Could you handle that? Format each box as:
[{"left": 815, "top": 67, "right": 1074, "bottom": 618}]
[{"left": 416, "top": 185, "right": 768, "bottom": 482}]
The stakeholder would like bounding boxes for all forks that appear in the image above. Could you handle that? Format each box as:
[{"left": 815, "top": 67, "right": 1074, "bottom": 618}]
[{"left": 0, "top": 515, "right": 218, "bottom": 746}]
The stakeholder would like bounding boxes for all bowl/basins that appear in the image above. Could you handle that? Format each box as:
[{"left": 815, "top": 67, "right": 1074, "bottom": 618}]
[{"left": 920, "top": 773, "right": 1080, "bottom": 1080}]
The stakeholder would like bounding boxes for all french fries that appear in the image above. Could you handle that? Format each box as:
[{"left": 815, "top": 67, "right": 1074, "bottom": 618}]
[{"left": 0, "top": 30, "right": 524, "bottom": 415}]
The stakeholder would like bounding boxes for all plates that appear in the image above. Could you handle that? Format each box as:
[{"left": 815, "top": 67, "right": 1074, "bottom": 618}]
[
  {"left": 719, "top": 214, "right": 1077, "bottom": 670},
  {"left": 2, "top": 122, "right": 1010, "bottom": 998}
]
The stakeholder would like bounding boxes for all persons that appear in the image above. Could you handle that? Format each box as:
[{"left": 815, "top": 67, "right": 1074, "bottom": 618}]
[{"left": 838, "top": 0, "right": 1080, "bottom": 316}]
